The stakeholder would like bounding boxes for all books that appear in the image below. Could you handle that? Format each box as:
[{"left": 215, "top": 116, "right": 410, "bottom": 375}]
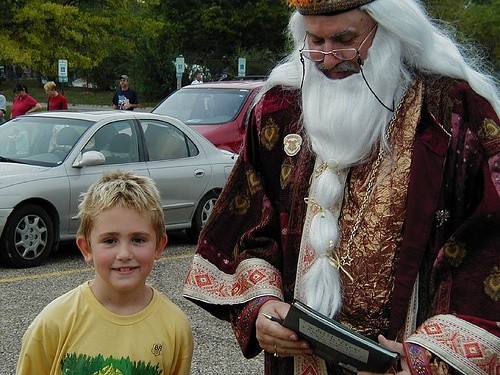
[{"left": 280, "top": 299, "right": 400, "bottom": 374}]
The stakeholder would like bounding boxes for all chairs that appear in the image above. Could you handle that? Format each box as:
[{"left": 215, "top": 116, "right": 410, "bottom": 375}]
[
  {"left": 106, "top": 133, "right": 133, "bottom": 163},
  {"left": 53, "top": 127, "right": 81, "bottom": 159}
]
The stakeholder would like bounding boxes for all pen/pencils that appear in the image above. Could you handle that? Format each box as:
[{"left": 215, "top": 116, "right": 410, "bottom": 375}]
[{"left": 262, "top": 313, "right": 282, "bottom": 324}]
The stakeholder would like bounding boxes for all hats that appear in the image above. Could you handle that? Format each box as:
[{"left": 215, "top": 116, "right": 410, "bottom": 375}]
[
  {"left": 120, "top": 75, "right": 128, "bottom": 80},
  {"left": 293, "top": 0, "right": 376, "bottom": 16}
]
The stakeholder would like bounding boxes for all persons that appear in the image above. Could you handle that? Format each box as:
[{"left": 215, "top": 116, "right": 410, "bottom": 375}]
[
  {"left": 179, "top": 0, "right": 500, "bottom": 375},
  {"left": 15, "top": 172, "right": 194, "bottom": 375},
  {"left": 0, "top": 94, "right": 6, "bottom": 126},
  {"left": 190, "top": 70, "right": 203, "bottom": 85},
  {"left": 10, "top": 84, "right": 42, "bottom": 120},
  {"left": 112, "top": 75, "right": 140, "bottom": 111},
  {"left": 44, "top": 81, "right": 67, "bottom": 111}
]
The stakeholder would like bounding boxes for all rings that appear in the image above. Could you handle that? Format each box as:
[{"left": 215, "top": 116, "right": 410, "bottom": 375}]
[{"left": 272, "top": 345, "right": 279, "bottom": 356}]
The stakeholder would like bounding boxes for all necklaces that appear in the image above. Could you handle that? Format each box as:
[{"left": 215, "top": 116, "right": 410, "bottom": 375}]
[{"left": 338, "top": 79, "right": 408, "bottom": 267}]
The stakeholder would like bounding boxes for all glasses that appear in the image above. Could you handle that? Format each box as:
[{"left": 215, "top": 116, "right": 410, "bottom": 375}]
[{"left": 299, "top": 22, "right": 378, "bottom": 62}]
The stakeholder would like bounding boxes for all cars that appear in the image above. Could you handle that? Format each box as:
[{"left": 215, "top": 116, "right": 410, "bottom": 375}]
[
  {"left": 69, "top": 76, "right": 97, "bottom": 88},
  {"left": 0, "top": 108, "right": 241, "bottom": 267},
  {"left": 148, "top": 75, "right": 266, "bottom": 154}
]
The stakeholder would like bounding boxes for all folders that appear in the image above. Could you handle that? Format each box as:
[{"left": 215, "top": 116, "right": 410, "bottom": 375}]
[{"left": 283, "top": 299, "right": 399, "bottom": 374}]
